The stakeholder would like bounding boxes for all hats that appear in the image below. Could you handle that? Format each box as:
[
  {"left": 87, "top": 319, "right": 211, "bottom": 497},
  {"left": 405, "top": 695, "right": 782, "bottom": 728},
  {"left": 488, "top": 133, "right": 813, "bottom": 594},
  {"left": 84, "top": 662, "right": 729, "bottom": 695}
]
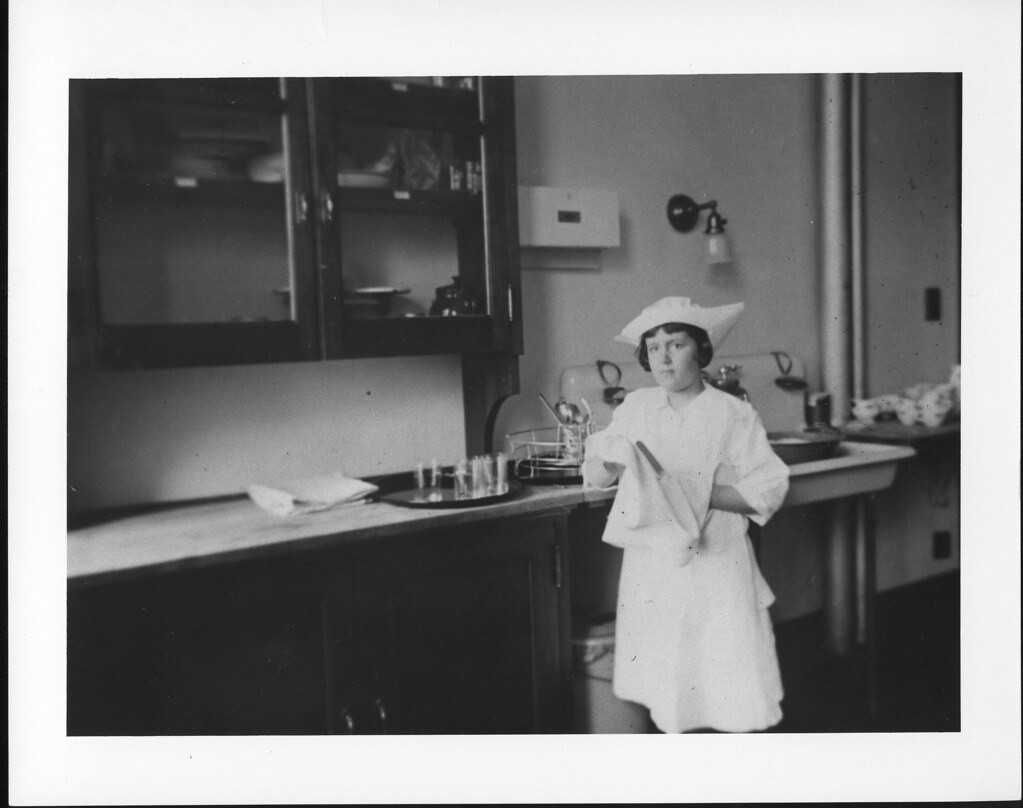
[{"left": 613, "top": 295, "right": 745, "bottom": 350}]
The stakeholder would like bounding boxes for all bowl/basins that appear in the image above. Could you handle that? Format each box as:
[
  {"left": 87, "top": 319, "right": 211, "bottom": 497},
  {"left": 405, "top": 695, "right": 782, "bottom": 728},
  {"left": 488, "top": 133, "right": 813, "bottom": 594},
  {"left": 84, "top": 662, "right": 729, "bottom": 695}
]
[
  {"left": 759, "top": 427, "right": 844, "bottom": 467},
  {"left": 274, "top": 285, "right": 413, "bottom": 316}
]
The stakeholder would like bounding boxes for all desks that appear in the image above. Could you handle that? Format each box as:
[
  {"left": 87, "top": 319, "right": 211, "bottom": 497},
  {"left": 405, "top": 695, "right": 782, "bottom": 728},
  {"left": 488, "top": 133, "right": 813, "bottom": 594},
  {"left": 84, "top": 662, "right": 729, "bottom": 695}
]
[{"left": 848, "top": 414, "right": 961, "bottom": 470}]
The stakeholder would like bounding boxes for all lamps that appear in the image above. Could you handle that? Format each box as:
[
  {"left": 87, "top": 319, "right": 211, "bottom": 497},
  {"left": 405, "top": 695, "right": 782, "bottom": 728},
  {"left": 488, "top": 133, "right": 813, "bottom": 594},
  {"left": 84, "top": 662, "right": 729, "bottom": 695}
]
[{"left": 666, "top": 192, "right": 732, "bottom": 266}]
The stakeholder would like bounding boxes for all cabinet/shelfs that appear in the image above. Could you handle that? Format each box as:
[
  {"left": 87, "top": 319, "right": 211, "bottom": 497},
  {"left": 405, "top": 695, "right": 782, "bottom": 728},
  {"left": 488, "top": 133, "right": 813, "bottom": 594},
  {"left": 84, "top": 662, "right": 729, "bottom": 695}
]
[
  {"left": 66, "top": 76, "right": 523, "bottom": 459},
  {"left": 67, "top": 507, "right": 576, "bottom": 736}
]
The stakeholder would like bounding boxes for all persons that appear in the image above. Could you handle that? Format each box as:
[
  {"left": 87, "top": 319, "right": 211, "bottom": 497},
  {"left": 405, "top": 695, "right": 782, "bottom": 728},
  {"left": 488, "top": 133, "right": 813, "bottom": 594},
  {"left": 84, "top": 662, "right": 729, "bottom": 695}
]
[{"left": 580, "top": 295, "right": 790, "bottom": 733}]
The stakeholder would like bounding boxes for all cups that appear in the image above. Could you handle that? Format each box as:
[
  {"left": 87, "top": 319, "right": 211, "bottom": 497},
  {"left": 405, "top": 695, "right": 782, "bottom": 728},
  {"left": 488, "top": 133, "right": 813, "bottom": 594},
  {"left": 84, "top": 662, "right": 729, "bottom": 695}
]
[
  {"left": 852, "top": 379, "right": 961, "bottom": 429},
  {"left": 414, "top": 452, "right": 513, "bottom": 501}
]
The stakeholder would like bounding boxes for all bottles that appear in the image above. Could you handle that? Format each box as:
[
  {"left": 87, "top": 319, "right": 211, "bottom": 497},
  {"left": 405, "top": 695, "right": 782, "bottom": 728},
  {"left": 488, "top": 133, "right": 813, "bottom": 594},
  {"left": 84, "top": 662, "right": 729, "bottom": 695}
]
[{"left": 429, "top": 275, "right": 485, "bottom": 316}]
